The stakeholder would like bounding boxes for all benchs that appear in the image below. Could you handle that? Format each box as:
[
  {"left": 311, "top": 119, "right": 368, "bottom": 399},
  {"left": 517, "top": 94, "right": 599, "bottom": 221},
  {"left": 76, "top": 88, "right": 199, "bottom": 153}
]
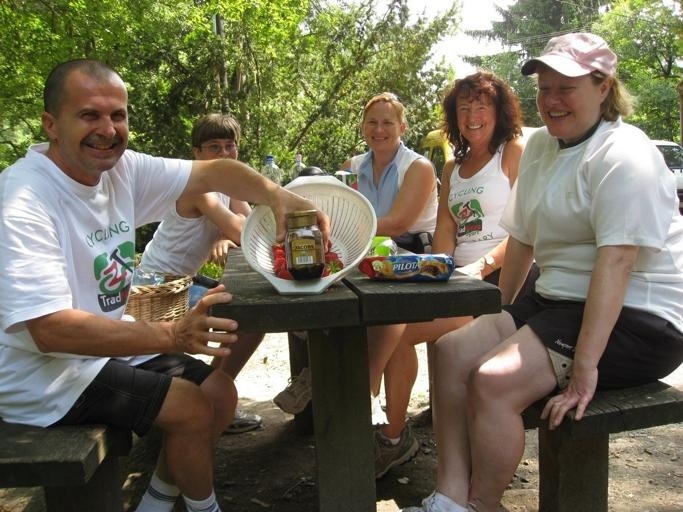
[
  {"left": 519, "top": 380, "right": 683, "bottom": 511},
  {"left": 0, "top": 417, "right": 133, "bottom": 512}
]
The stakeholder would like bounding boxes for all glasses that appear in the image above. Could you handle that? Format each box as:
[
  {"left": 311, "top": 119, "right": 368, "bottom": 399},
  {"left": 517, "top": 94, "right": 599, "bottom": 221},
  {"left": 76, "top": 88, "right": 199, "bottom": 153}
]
[{"left": 195, "top": 141, "right": 238, "bottom": 154}]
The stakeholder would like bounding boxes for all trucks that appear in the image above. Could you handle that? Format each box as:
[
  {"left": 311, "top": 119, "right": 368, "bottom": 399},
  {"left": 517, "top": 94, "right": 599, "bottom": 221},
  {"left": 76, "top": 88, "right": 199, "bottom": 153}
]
[{"left": 417, "top": 127, "right": 538, "bottom": 202}]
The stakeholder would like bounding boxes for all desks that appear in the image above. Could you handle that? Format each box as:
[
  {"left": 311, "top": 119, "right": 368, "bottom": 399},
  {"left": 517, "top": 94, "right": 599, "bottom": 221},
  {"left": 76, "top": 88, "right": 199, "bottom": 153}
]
[{"left": 208, "top": 246, "right": 502, "bottom": 512}]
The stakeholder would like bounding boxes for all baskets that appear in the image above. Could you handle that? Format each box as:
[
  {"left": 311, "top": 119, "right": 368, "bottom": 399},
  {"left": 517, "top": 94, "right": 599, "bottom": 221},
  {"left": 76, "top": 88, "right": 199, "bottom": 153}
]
[
  {"left": 239, "top": 174, "right": 378, "bottom": 295},
  {"left": 126, "top": 275, "right": 193, "bottom": 326}
]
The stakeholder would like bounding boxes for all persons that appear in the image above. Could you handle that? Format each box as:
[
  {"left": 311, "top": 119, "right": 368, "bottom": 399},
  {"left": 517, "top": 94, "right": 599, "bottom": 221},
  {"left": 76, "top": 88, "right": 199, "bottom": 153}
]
[
  {"left": 0, "top": 60, "right": 331, "bottom": 512},
  {"left": 392, "top": 31, "right": 683, "bottom": 512},
  {"left": 132, "top": 113, "right": 264, "bottom": 435},
  {"left": 371, "top": 71, "right": 525, "bottom": 480},
  {"left": 273, "top": 94, "right": 437, "bottom": 416}
]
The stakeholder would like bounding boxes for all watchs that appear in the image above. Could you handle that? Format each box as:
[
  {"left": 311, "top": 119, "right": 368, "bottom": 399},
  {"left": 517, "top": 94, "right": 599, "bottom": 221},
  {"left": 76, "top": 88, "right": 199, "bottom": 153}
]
[{"left": 483, "top": 254, "right": 499, "bottom": 273}]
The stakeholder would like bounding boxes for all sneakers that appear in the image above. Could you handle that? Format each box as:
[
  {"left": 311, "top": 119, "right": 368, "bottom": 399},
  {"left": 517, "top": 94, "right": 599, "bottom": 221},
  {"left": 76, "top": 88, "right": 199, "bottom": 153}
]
[
  {"left": 224, "top": 409, "right": 263, "bottom": 435},
  {"left": 376, "top": 423, "right": 419, "bottom": 479},
  {"left": 273, "top": 365, "right": 313, "bottom": 414}
]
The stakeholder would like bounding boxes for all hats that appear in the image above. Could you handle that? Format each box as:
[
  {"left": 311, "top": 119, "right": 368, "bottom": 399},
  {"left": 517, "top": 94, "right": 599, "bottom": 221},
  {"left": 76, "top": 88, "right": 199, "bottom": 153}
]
[{"left": 522, "top": 34, "right": 618, "bottom": 79}]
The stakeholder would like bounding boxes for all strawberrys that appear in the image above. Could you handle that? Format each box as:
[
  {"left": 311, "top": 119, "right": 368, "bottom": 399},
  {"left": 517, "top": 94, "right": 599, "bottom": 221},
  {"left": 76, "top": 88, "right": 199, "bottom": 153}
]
[{"left": 273, "top": 239, "right": 344, "bottom": 280}]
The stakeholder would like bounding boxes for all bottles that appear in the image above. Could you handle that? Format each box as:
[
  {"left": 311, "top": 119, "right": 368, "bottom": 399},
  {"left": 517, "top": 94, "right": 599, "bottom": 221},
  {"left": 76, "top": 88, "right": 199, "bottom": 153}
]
[
  {"left": 260, "top": 155, "right": 281, "bottom": 186},
  {"left": 289, "top": 153, "right": 308, "bottom": 182}
]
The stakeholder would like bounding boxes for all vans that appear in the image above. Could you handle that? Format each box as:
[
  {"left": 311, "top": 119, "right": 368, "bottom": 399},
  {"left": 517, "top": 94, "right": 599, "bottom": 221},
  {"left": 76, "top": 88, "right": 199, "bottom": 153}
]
[{"left": 651, "top": 138, "right": 682, "bottom": 210}]
